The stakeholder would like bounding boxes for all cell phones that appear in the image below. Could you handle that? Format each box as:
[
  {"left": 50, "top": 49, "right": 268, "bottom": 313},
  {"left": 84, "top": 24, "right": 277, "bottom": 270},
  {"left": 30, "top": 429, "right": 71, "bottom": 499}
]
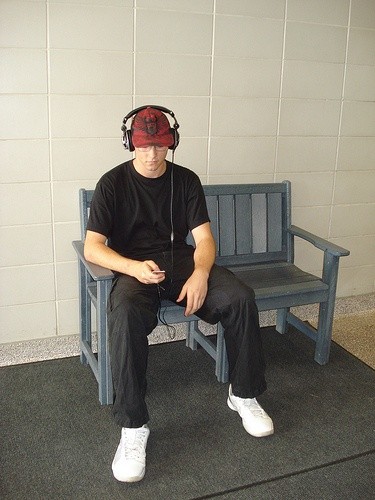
[{"left": 152, "top": 271, "right": 165, "bottom": 274}]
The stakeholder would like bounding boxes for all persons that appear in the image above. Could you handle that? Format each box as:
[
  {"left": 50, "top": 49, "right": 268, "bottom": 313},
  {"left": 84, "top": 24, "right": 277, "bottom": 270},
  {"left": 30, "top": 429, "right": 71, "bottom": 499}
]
[{"left": 83, "top": 105, "right": 274, "bottom": 483}]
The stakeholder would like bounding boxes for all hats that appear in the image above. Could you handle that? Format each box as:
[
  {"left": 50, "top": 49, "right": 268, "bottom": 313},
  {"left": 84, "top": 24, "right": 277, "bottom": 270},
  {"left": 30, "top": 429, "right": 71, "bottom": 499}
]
[{"left": 131, "top": 107, "right": 174, "bottom": 147}]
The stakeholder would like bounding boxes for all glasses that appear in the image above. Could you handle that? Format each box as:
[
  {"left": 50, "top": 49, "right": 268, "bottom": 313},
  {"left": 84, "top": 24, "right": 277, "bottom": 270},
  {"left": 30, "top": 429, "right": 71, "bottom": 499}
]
[{"left": 136, "top": 147, "right": 168, "bottom": 151}]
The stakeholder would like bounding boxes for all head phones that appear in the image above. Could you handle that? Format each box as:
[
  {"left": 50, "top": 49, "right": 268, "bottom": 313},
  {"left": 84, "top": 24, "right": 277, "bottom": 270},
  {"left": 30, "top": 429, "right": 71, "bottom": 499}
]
[{"left": 121, "top": 105, "right": 179, "bottom": 152}]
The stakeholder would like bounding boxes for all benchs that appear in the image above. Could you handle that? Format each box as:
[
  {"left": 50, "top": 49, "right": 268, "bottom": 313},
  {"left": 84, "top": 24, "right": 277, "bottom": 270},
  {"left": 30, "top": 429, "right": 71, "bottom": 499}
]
[{"left": 72, "top": 180, "right": 350, "bottom": 405}]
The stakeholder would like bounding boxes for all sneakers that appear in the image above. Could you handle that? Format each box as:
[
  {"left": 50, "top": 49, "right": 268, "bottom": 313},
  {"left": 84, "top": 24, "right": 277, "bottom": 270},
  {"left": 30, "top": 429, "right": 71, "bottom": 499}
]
[
  {"left": 226, "top": 383, "right": 275, "bottom": 437},
  {"left": 111, "top": 424, "right": 150, "bottom": 483}
]
